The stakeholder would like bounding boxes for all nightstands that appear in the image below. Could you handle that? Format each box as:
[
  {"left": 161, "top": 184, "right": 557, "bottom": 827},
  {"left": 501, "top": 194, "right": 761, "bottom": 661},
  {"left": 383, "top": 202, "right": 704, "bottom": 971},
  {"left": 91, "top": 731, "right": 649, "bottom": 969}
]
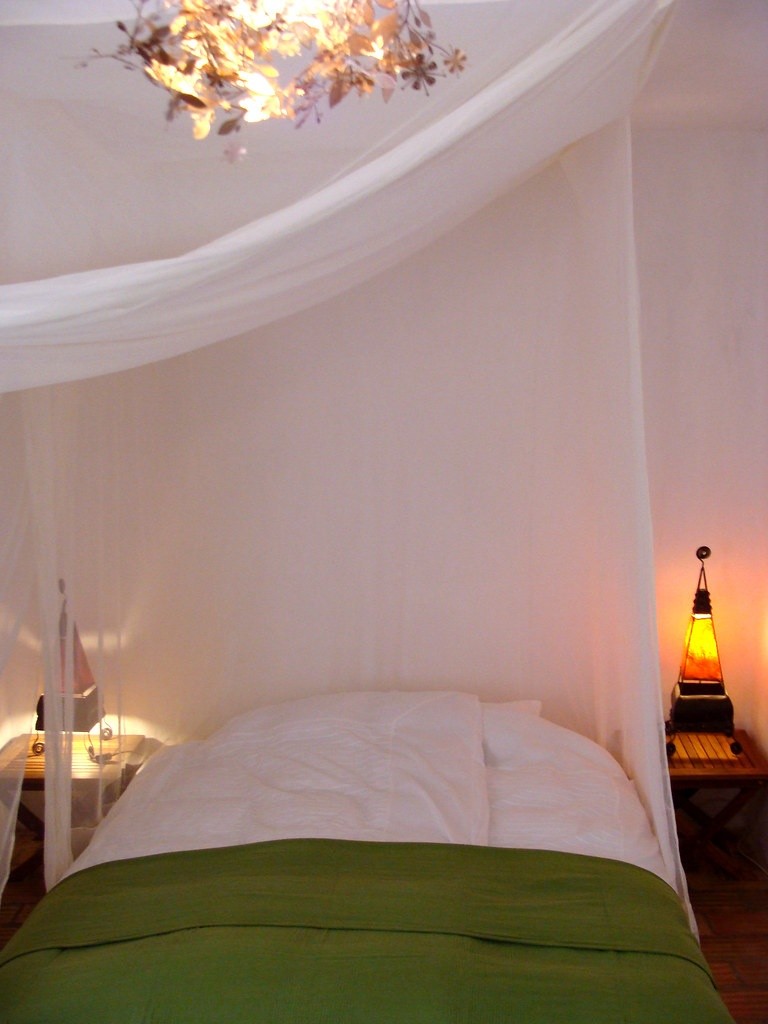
[
  {"left": 666, "top": 729, "right": 768, "bottom": 879},
  {"left": 0, "top": 734, "right": 145, "bottom": 882}
]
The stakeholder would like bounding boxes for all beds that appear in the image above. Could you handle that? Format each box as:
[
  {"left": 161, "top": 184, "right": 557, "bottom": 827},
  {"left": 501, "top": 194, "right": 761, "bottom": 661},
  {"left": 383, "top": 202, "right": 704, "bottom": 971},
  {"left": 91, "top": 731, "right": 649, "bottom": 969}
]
[{"left": 1, "top": 693, "right": 735, "bottom": 1024}]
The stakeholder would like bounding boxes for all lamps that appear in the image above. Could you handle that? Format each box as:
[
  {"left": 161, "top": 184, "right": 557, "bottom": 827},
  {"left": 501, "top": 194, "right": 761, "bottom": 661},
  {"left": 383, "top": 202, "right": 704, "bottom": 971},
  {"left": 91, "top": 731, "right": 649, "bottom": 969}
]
[
  {"left": 99, "top": 1, "right": 468, "bottom": 140},
  {"left": 666, "top": 545, "right": 742, "bottom": 758},
  {"left": 32, "top": 579, "right": 147, "bottom": 763}
]
